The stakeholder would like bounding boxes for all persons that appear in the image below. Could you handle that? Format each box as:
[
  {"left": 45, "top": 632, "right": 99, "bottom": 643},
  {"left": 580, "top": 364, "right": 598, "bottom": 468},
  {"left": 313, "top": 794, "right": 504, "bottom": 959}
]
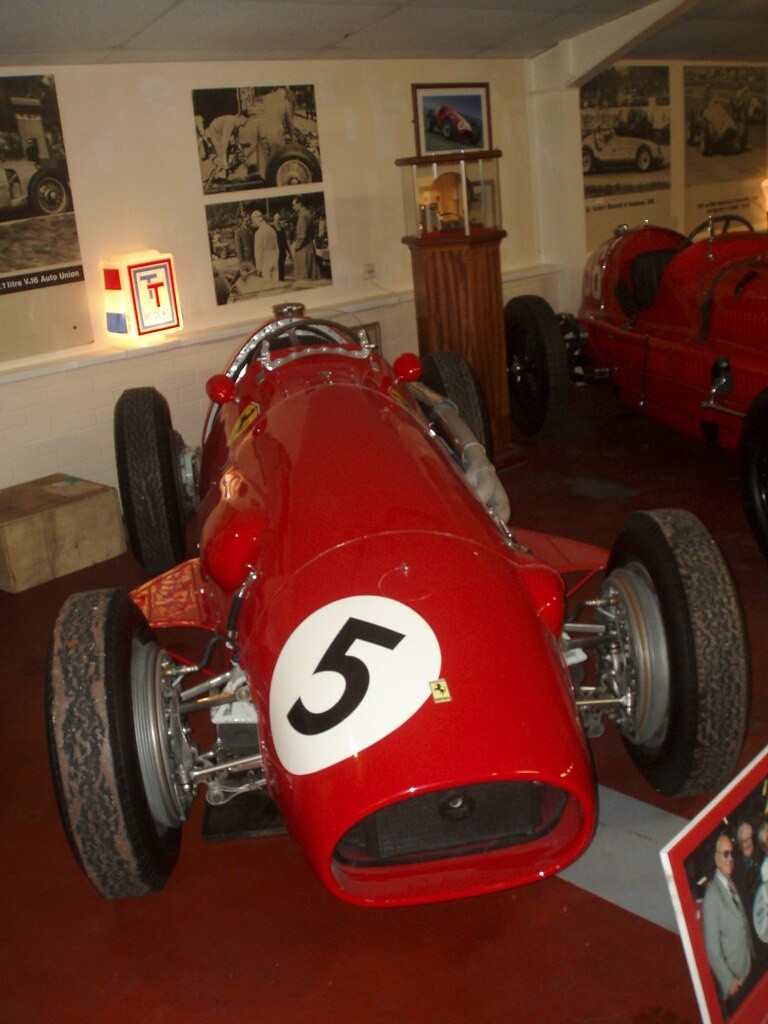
[
  {"left": 193, "top": 85, "right": 316, "bottom": 189},
  {"left": 580, "top": 70, "right": 749, "bottom": 151},
  {"left": 701, "top": 823, "right": 768, "bottom": 1016},
  {"left": 208, "top": 196, "right": 328, "bottom": 305}
]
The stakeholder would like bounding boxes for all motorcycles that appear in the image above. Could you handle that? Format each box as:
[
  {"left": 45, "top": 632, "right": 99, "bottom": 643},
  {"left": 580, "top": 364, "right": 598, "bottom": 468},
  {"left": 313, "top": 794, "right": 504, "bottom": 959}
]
[
  {"left": 686, "top": 102, "right": 747, "bottom": 155},
  {"left": 1, "top": 159, "right": 71, "bottom": 216},
  {"left": 505, "top": 214, "right": 767, "bottom": 561},
  {"left": 426, "top": 106, "right": 480, "bottom": 146},
  {"left": 46, "top": 302, "right": 748, "bottom": 909},
  {"left": 582, "top": 130, "right": 664, "bottom": 175}
]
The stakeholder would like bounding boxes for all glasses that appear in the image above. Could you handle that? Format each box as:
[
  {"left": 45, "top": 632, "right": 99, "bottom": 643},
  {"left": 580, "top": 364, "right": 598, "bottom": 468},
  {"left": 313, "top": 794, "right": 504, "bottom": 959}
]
[{"left": 716, "top": 850, "right": 733, "bottom": 857}]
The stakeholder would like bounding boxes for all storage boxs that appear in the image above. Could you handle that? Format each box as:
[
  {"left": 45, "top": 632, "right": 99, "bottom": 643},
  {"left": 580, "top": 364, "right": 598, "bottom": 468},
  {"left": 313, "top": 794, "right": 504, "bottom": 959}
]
[
  {"left": 0, "top": 472, "right": 129, "bottom": 594},
  {"left": 397, "top": 149, "right": 502, "bottom": 238}
]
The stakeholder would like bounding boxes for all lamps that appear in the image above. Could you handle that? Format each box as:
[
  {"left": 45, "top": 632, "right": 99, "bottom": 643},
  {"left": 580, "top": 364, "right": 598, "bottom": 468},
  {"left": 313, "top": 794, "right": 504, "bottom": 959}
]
[{"left": 102, "top": 250, "right": 185, "bottom": 347}]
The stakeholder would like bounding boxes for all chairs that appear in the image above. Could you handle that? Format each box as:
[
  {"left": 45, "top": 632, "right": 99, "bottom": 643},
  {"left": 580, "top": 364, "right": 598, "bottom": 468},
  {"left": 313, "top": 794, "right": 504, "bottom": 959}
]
[{"left": 631, "top": 248, "right": 677, "bottom": 312}]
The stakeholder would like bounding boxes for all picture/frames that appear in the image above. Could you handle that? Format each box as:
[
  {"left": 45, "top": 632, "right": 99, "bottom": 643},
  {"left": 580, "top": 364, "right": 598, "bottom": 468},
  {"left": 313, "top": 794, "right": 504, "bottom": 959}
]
[
  {"left": 658, "top": 743, "right": 768, "bottom": 1024},
  {"left": 411, "top": 81, "right": 493, "bottom": 156}
]
